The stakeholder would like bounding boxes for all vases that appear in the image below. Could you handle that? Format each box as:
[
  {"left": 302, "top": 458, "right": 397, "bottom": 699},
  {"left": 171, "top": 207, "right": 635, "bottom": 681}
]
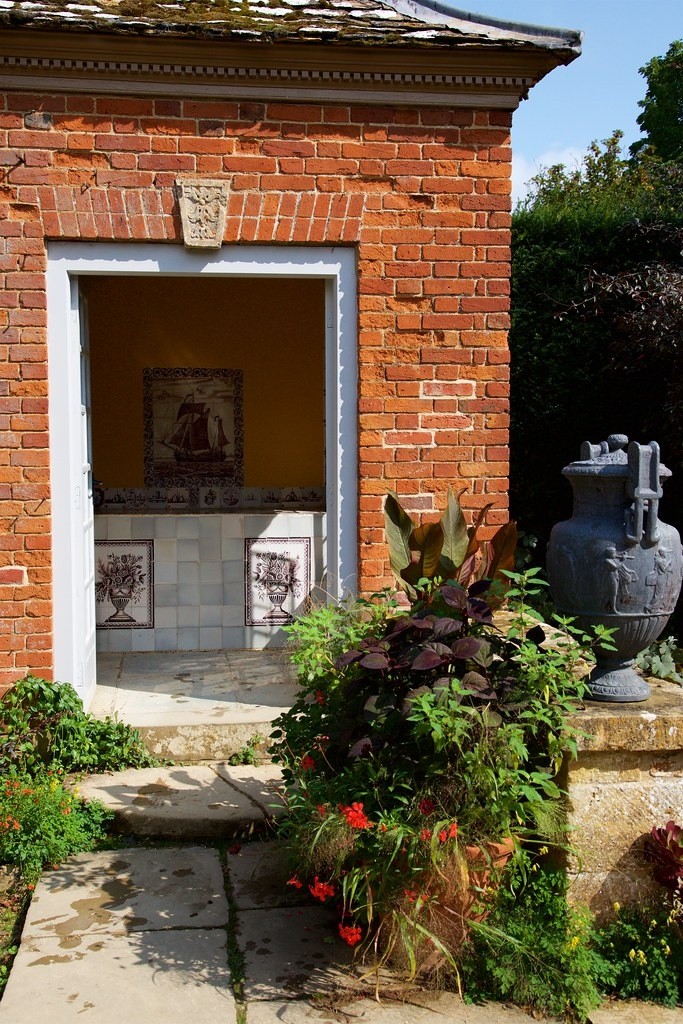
[{"left": 374, "top": 834, "right": 520, "bottom": 973}]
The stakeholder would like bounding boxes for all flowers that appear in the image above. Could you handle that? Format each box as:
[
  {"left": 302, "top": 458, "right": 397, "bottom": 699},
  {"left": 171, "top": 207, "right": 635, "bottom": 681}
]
[{"left": 223, "top": 487, "right": 618, "bottom": 1003}]
